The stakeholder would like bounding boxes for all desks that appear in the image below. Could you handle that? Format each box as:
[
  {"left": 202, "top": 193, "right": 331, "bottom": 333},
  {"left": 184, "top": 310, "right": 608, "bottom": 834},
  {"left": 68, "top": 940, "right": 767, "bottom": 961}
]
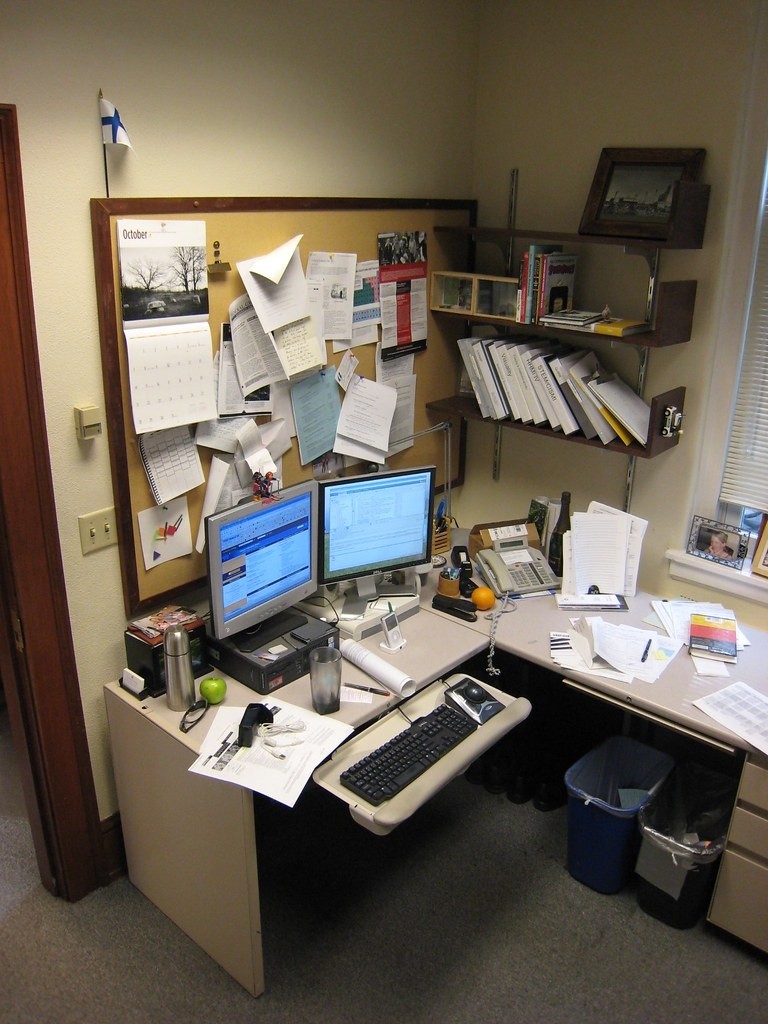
[{"left": 74, "top": 527, "right": 768, "bottom": 998}]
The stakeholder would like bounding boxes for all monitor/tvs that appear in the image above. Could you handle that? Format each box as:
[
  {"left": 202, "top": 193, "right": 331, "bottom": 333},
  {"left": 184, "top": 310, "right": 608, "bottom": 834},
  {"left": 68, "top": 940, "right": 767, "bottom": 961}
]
[
  {"left": 203, "top": 479, "right": 318, "bottom": 652},
  {"left": 317, "top": 465, "right": 438, "bottom": 620}
]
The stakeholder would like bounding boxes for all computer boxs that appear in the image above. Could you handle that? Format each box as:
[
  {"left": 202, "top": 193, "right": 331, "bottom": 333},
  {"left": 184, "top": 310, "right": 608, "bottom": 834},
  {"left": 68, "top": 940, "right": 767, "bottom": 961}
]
[{"left": 200, "top": 590, "right": 422, "bottom": 696}]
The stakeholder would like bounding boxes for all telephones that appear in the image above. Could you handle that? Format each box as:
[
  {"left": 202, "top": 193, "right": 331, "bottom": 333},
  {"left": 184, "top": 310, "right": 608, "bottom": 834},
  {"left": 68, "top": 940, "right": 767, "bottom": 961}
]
[{"left": 474, "top": 546, "right": 561, "bottom": 597}]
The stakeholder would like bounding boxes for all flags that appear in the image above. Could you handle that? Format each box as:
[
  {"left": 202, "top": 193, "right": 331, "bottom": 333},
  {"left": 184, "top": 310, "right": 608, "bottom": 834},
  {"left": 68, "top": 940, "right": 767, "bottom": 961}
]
[{"left": 100, "top": 100, "right": 136, "bottom": 151}]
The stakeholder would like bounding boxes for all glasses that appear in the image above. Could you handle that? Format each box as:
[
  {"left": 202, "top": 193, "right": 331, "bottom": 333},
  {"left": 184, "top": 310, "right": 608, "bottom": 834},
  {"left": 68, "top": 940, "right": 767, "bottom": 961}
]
[{"left": 181, "top": 699, "right": 211, "bottom": 733}]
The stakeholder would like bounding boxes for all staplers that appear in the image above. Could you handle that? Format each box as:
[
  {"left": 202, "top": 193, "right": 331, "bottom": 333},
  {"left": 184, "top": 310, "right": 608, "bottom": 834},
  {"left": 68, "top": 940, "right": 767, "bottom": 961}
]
[{"left": 430, "top": 594, "right": 478, "bottom": 622}]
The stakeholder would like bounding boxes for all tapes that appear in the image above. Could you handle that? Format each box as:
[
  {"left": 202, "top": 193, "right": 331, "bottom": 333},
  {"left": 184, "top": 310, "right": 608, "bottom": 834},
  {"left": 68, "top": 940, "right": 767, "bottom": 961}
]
[{"left": 459, "top": 552, "right": 466, "bottom": 562}]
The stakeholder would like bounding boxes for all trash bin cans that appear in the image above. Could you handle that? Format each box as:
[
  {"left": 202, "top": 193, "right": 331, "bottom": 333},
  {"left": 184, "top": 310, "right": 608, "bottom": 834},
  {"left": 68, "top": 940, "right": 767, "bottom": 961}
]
[
  {"left": 565, "top": 735, "right": 671, "bottom": 895},
  {"left": 634, "top": 758, "right": 736, "bottom": 931}
]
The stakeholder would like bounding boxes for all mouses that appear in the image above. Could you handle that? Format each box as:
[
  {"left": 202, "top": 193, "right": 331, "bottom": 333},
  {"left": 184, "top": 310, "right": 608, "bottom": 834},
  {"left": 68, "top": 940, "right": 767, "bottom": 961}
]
[{"left": 464, "top": 684, "right": 488, "bottom": 703}]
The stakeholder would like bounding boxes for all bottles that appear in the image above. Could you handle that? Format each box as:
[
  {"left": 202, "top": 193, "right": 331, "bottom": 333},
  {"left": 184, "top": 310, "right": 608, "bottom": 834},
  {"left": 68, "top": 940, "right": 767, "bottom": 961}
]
[{"left": 548, "top": 491, "right": 572, "bottom": 578}]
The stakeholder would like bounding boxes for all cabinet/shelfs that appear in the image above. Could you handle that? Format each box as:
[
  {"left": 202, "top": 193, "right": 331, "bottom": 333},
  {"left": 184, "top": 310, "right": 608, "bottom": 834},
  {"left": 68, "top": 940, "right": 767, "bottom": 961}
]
[
  {"left": 426, "top": 179, "right": 713, "bottom": 459},
  {"left": 705, "top": 753, "right": 768, "bottom": 957}
]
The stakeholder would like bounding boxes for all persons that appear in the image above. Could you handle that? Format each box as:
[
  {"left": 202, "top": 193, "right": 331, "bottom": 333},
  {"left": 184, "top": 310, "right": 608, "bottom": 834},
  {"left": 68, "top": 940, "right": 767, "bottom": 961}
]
[
  {"left": 379, "top": 232, "right": 427, "bottom": 266},
  {"left": 149, "top": 609, "right": 191, "bottom": 630},
  {"left": 702, "top": 531, "right": 733, "bottom": 561},
  {"left": 319, "top": 455, "right": 329, "bottom": 473},
  {"left": 263, "top": 472, "right": 279, "bottom": 497},
  {"left": 252, "top": 472, "right": 263, "bottom": 502}
]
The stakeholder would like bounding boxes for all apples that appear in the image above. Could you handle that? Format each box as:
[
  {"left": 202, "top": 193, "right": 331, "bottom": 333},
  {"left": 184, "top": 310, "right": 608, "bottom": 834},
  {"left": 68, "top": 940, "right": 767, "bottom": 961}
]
[{"left": 200, "top": 677, "right": 227, "bottom": 705}]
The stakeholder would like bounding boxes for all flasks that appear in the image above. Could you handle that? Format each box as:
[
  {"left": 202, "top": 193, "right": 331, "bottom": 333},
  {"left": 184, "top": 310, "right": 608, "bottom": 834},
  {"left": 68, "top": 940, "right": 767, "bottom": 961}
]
[{"left": 163, "top": 626, "right": 196, "bottom": 712}]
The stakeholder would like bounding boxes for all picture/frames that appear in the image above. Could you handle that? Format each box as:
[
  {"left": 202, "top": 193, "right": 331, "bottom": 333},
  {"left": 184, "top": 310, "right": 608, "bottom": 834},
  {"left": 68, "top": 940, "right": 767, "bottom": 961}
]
[
  {"left": 577, "top": 148, "right": 708, "bottom": 242},
  {"left": 684, "top": 515, "right": 751, "bottom": 570},
  {"left": 749, "top": 521, "right": 768, "bottom": 578}
]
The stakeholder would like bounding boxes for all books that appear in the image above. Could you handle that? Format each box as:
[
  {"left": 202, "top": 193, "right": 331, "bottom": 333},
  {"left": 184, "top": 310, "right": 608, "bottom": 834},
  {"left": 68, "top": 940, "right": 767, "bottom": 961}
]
[
  {"left": 555, "top": 594, "right": 629, "bottom": 612},
  {"left": 688, "top": 614, "right": 737, "bottom": 665},
  {"left": 562, "top": 501, "right": 649, "bottom": 596},
  {"left": 457, "top": 335, "right": 650, "bottom": 447},
  {"left": 516, "top": 244, "right": 646, "bottom": 337}
]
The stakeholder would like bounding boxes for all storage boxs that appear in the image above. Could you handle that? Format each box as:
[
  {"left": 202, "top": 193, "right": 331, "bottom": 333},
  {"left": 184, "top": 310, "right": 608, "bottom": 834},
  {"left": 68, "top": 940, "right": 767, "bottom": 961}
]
[{"left": 466, "top": 518, "right": 541, "bottom": 560}]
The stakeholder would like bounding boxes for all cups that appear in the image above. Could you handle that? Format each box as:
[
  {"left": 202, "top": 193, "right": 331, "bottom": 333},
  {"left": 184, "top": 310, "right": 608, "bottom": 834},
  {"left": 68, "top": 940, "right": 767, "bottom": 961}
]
[{"left": 309, "top": 647, "right": 343, "bottom": 715}]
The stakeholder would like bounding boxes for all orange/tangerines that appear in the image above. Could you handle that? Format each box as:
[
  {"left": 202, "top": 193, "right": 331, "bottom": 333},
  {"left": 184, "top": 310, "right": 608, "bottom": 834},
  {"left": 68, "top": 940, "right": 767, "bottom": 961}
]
[{"left": 471, "top": 586, "right": 495, "bottom": 610}]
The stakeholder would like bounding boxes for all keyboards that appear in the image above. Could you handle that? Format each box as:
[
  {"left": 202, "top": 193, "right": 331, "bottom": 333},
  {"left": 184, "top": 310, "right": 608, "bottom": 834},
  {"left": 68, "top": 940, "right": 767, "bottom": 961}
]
[{"left": 340, "top": 704, "right": 478, "bottom": 807}]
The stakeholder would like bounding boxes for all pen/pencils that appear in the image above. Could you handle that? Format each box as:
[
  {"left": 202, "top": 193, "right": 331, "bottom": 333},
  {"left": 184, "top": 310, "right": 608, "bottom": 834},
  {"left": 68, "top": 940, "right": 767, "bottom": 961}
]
[
  {"left": 641, "top": 638, "right": 653, "bottom": 663},
  {"left": 343, "top": 681, "right": 391, "bottom": 697},
  {"left": 434, "top": 498, "right": 445, "bottom": 524},
  {"left": 436, "top": 520, "right": 444, "bottom": 534},
  {"left": 280, "top": 634, "right": 298, "bottom": 651}
]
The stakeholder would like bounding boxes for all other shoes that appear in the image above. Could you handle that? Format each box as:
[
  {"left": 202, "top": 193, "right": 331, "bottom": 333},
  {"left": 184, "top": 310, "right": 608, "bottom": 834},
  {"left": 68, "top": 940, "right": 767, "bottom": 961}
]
[{"left": 462, "top": 734, "right": 568, "bottom": 812}]
